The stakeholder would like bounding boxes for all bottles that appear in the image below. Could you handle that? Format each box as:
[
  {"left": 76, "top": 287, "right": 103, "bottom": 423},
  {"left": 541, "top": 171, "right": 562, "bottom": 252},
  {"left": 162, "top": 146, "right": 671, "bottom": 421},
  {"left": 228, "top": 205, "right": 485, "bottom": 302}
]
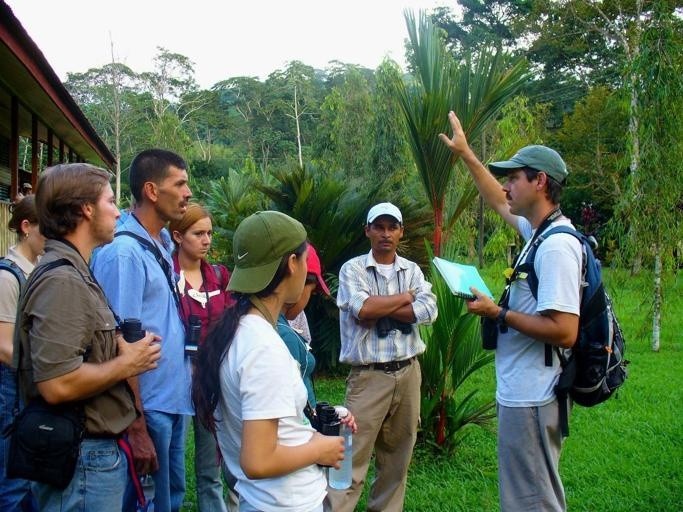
[{"left": 326, "top": 407, "right": 352, "bottom": 491}]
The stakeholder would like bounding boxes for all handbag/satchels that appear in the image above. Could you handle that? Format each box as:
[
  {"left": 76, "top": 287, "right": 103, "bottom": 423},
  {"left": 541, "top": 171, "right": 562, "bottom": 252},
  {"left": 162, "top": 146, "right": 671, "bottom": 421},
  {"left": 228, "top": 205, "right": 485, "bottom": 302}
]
[{"left": 6, "top": 401, "right": 80, "bottom": 491}]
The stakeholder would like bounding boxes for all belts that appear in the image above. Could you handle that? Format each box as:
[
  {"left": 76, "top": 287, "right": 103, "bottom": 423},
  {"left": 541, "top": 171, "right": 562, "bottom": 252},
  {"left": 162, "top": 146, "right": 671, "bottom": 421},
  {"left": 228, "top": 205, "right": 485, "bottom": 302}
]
[{"left": 363, "top": 357, "right": 415, "bottom": 371}]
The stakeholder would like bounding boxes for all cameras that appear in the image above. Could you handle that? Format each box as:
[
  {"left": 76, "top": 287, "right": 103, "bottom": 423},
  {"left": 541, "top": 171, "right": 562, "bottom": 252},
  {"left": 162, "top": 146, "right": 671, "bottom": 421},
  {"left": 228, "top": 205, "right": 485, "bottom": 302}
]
[{"left": 480, "top": 314, "right": 498, "bottom": 350}]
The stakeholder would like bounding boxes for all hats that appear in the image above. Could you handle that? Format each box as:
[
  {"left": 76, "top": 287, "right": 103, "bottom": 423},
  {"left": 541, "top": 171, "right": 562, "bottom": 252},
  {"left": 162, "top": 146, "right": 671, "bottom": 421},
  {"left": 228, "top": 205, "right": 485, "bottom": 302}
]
[
  {"left": 367, "top": 203, "right": 402, "bottom": 225},
  {"left": 488, "top": 145, "right": 570, "bottom": 188},
  {"left": 225, "top": 211, "right": 307, "bottom": 294},
  {"left": 306, "top": 243, "right": 330, "bottom": 296}
]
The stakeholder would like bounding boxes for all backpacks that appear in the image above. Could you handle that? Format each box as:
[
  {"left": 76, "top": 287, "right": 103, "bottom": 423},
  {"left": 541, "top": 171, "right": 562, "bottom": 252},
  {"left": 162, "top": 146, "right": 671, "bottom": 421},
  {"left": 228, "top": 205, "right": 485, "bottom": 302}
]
[{"left": 525, "top": 226, "right": 627, "bottom": 437}]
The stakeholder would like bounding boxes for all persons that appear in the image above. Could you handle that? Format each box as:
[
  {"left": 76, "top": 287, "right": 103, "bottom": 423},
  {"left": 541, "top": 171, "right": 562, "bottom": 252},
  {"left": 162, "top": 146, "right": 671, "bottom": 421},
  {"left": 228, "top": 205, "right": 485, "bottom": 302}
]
[
  {"left": 323, "top": 201, "right": 438, "bottom": 512},
  {"left": 0, "top": 149, "right": 357, "bottom": 512},
  {"left": 438, "top": 110, "right": 585, "bottom": 512}
]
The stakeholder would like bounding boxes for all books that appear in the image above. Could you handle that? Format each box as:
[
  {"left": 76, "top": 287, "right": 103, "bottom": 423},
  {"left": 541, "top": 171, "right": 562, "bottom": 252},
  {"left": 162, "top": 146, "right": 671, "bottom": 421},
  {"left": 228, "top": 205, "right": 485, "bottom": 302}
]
[{"left": 431, "top": 256, "right": 495, "bottom": 302}]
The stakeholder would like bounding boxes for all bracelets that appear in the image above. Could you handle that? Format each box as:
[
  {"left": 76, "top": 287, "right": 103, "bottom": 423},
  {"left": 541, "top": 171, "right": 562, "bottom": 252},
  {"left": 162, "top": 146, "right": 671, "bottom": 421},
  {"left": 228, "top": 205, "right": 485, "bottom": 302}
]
[{"left": 496, "top": 307, "right": 509, "bottom": 322}]
